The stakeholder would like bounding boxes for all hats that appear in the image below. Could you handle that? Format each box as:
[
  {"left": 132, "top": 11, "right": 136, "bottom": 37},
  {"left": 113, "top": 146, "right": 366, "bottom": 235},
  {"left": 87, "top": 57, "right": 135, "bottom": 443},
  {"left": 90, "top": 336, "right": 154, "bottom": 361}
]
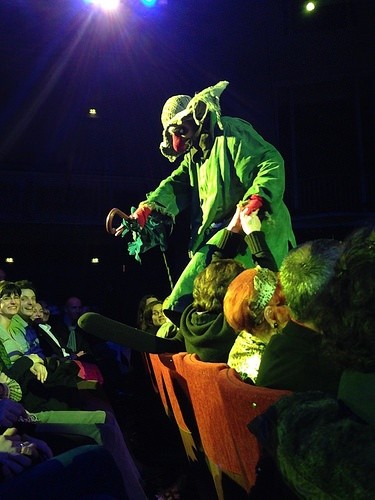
[{"left": 160, "top": 81, "right": 229, "bottom": 162}]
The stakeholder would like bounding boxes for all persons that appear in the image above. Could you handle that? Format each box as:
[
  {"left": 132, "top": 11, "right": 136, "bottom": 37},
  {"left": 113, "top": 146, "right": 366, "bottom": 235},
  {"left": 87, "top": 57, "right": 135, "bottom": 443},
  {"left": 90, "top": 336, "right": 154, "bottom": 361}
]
[
  {"left": 0, "top": 225, "right": 375, "bottom": 500},
  {"left": 77, "top": 81, "right": 298, "bottom": 350}
]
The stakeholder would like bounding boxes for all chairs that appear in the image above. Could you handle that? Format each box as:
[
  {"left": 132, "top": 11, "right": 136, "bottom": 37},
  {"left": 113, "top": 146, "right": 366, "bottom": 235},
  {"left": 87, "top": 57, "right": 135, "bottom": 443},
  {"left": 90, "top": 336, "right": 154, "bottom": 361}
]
[{"left": 149, "top": 351, "right": 293, "bottom": 500}]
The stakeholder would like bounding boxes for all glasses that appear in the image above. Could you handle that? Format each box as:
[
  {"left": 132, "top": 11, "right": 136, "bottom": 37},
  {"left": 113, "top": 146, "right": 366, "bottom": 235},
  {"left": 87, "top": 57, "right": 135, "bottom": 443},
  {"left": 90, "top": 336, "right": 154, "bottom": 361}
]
[{"left": 0, "top": 296, "right": 20, "bottom": 301}]
